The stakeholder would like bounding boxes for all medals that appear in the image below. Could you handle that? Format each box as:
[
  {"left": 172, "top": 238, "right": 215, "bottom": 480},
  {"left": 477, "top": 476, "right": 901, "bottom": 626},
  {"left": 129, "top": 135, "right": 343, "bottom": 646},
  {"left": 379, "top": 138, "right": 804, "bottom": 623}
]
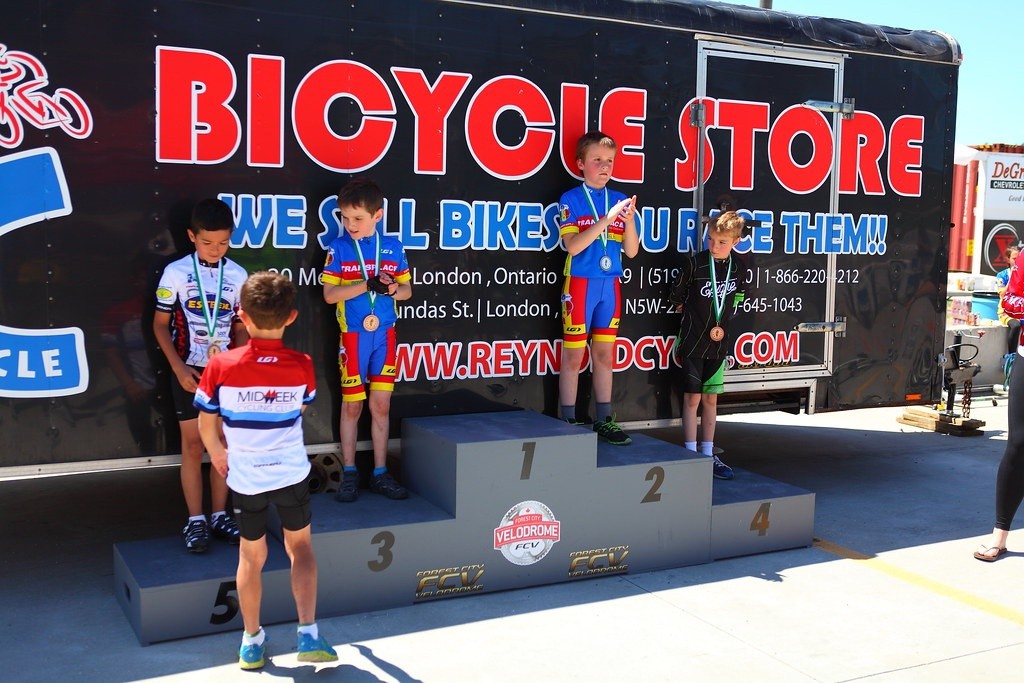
[
  {"left": 710, "top": 326, "right": 724, "bottom": 341},
  {"left": 207, "top": 345, "right": 222, "bottom": 360},
  {"left": 600, "top": 256, "right": 612, "bottom": 272},
  {"left": 363, "top": 314, "right": 380, "bottom": 332}
]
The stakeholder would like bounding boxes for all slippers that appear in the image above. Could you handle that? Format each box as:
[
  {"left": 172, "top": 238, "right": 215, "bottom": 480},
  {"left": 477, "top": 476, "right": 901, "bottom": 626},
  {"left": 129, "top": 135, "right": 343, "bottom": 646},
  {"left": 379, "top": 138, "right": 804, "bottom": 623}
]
[{"left": 973, "top": 543, "right": 1007, "bottom": 561}]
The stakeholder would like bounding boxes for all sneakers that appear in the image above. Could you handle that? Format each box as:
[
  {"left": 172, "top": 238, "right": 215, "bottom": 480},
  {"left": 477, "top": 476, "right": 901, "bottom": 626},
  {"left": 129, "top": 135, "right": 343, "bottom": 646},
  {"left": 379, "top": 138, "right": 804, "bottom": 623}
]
[
  {"left": 296, "top": 630, "right": 338, "bottom": 662},
  {"left": 710, "top": 453, "right": 734, "bottom": 479},
  {"left": 560, "top": 416, "right": 584, "bottom": 426},
  {"left": 593, "top": 412, "right": 632, "bottom": 444},
  {"left": 239, "top": 630, "right": 269, "bottom": 669},
  {"left": 182, "top": 520, "right": 209, "bottom": 553},
  {"left": 209, "top": 514, "right": 240, "bottom": 544},
  {"left": 334, "top": 470, "right": 360, "bottom": 500},
  {"left": 371, "top": 471, "right": 408, "bottom": 499}
]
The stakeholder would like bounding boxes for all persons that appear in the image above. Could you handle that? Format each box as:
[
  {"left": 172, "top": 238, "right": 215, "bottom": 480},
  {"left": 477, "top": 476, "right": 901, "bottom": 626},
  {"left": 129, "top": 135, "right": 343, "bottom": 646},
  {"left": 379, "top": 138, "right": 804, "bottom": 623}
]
[
  {"left": 320, "top": 178, "right": 413, "bottom": 504},
  {"left": 191, "top": 270, "right": 338, "bottom": 669},
  {"left": 677, "top": 209, "right": 745, "bottom": 480},
  {"left": 152, "top": 196, "right": 251, "bottom": 555},
  {"left": 973, "top": 240, "right": 1024, "bottom": 562},
  {"left": 102, "top": 266, "right": 183, "bottom": 455},
  {"left": 557, "top": 132, "right": 639, "bottom": 445}
]
